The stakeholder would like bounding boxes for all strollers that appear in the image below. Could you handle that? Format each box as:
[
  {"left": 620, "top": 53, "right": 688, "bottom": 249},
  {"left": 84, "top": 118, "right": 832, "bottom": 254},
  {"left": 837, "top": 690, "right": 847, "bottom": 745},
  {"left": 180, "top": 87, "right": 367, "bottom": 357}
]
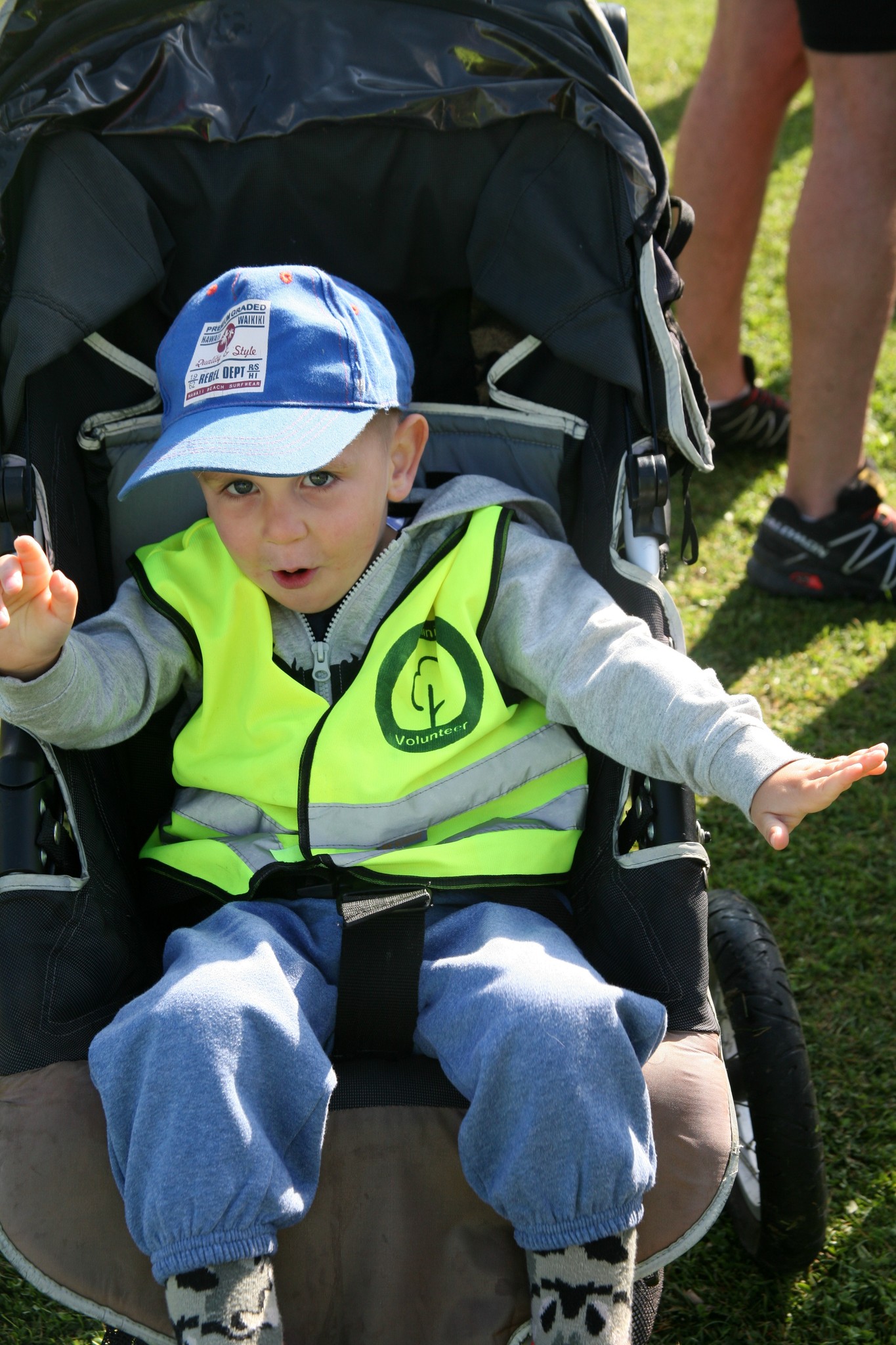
[{"left": 0, "top": 0, "right": 827, "bottom": 1345}]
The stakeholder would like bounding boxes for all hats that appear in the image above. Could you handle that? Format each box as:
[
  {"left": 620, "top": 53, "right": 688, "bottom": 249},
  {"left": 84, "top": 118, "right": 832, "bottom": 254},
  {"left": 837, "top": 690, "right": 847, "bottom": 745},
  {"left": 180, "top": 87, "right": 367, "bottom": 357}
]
[{"left": 117, "top": 264, "right": 415, "bottom": 500}]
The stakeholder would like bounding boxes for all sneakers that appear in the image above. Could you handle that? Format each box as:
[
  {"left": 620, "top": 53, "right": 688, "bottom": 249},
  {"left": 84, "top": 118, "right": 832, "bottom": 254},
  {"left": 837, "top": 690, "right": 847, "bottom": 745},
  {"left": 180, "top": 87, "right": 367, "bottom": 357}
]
[
  {"left": 705, "top": 354, "right": 791, "bottom": 453},
  {"left": 745, "top": 457, "right": 895, "bottom": 605}
]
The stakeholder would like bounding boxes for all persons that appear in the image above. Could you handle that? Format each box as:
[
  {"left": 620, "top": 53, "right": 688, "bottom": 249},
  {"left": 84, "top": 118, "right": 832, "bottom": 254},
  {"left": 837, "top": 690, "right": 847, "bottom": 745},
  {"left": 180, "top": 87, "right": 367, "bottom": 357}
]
[
  {"left": 660, "top": 1, "right": 895, "bottom": 606},
  {"left": 0, "top": 254, "right": 886, "bottom": 1344}
]
